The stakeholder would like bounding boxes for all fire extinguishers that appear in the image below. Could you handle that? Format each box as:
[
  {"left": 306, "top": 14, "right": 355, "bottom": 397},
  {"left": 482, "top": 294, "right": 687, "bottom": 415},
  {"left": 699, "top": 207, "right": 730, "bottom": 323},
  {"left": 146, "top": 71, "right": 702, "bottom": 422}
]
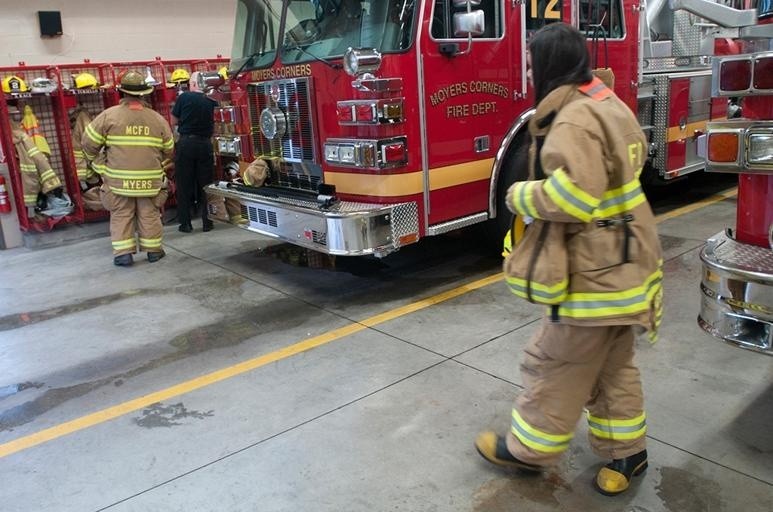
[{"left": 0, "top": 171, "right": 11, "bottom": 214}]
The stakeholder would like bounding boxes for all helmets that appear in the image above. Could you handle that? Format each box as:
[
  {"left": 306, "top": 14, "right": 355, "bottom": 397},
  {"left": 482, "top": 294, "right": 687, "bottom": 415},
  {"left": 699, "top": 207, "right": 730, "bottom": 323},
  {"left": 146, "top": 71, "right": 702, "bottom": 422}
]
[
  {"left": 74, "top": 74, "right": 100, "bottom": 88},
  {"left": 0, "top": 76, "right": 26, "bottom": 94},
  {"left": 116, "top": 71, "right": 153, "bottom": 96},
  {"left": 170, "top": 70, "right": 190, "bottom": 83},
  {"left": 501, "top": 211, "right": 526, "bottom": 259}
]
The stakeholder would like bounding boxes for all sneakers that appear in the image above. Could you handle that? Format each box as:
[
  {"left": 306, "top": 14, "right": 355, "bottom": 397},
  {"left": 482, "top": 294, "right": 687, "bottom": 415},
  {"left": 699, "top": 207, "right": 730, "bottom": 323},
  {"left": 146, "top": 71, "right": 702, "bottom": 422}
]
[
  {"left": 476, "top": 431, "right": 545, "bottom": 474},
  {"left": 201, "top": 218, "right": 215, "bottom": 232},
  {"left": 178, "top": 221, "right": 193, "bottom": 233},
  {"left": 595, "top": 448, "right": 646, "bottom": 494},
  {"left": 147, "top": 249, "right": 166, "bottom": 264},
  {"left": 112, "top": 254, "right": 134, "bottom": 267}
]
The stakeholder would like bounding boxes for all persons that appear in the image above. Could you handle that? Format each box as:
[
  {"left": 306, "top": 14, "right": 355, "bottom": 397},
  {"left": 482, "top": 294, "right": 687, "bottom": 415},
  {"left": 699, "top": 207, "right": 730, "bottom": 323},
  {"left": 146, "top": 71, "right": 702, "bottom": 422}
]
[
  {"left": 80, "top": 70, "right": 220, "bottom": 267},
  {"left": 475, "top": 22, "right": 663, "bottom": 494}
]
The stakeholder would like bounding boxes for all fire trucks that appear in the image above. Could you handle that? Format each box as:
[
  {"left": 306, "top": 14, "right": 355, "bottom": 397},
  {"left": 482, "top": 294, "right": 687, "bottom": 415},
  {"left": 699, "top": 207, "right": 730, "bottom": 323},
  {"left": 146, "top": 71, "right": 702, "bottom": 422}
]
[
  {"left": 197, "top": 1, "right": 722, "bottom": 270},
  {"left": 665, "top": 0, "right": 773, "bottom": 357}
]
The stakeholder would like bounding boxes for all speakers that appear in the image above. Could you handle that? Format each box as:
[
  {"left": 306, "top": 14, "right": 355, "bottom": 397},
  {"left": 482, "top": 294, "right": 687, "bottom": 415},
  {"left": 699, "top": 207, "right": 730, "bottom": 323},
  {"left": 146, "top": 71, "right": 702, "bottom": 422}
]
[{"left": 39, "top": 11, "right": 63, "bottom": 36}]
